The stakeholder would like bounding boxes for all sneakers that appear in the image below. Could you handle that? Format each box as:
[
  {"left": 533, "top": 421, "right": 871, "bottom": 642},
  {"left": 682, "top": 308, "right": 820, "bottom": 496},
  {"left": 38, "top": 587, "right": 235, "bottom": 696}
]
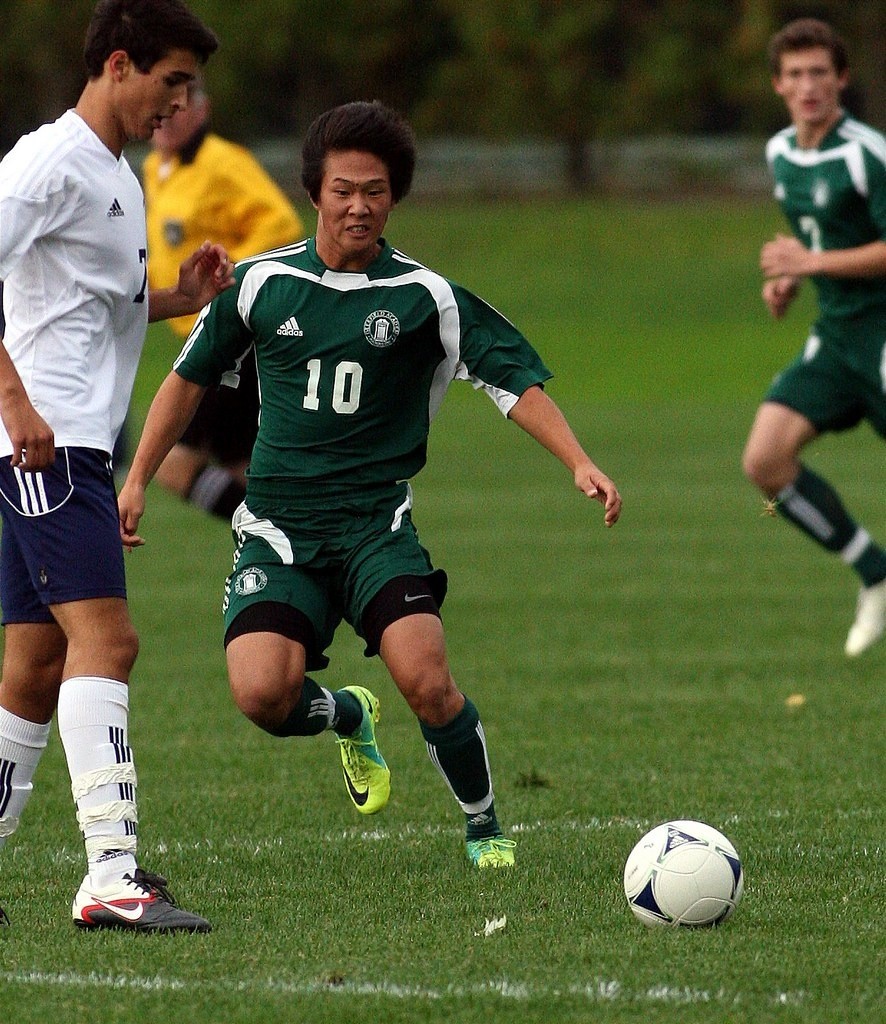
[
  {"left": 466, "top": 834, "right": 517, "bottom": 870},
  {"left": 71, "top": 869, "right": 212, "bottom": 933},
  {"left": 334, "top": 685, "right": 391, "bottom": 815},
  {"left": 842, "top": 579, "right": 886, "bottom": 656}
]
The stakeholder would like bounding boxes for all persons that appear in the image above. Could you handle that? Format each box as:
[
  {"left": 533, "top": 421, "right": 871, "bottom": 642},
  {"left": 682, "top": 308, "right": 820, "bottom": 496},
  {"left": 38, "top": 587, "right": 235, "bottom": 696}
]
[
  {"left": 117, "top": 102, "right": 621, "bottom": 869},
  {"left": 1, "top": 2, "right": 220, "bottom": 933},
  {"left": 142, "top": 66, "right": 305, "bottom": 516},
  {"left": 740, "top": 19, "right": 886, "bottom": 661}
]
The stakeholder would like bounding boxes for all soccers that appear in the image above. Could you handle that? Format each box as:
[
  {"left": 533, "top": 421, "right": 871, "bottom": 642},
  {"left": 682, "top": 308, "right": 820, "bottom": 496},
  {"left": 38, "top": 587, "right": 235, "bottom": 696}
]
[{"left": 624, "top": 819, "right": 744, "bottom": 928}]
